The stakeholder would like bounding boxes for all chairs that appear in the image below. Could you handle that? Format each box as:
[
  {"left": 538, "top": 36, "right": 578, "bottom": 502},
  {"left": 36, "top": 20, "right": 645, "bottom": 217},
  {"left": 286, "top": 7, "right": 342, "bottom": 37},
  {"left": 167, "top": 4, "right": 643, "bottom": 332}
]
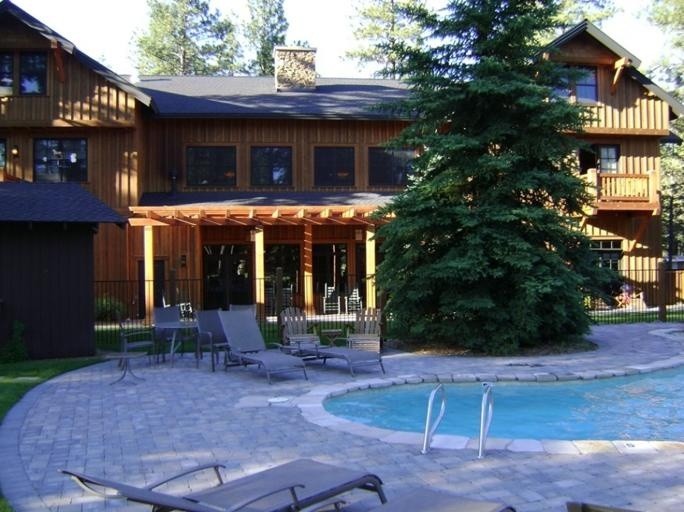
[
  {"left": 114, "top": 300, "right": 387, "bottom": 386},
  {"left": 56, "top": 456, "right": 388, "bottom": 510}
]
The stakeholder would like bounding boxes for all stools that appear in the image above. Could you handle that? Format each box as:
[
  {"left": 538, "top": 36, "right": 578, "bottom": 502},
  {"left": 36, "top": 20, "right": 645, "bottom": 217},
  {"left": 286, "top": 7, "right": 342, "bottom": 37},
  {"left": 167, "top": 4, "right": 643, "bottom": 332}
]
[{"left": 306, "top": 486, "right": 519, "bottom": 511}]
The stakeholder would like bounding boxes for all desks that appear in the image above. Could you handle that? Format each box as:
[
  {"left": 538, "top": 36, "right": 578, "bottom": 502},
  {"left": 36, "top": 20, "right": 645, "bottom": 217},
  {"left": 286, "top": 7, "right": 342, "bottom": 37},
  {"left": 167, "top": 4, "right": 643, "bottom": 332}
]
[{"left": 104, "top": 351, "right": 149, "bottom": 388}]
